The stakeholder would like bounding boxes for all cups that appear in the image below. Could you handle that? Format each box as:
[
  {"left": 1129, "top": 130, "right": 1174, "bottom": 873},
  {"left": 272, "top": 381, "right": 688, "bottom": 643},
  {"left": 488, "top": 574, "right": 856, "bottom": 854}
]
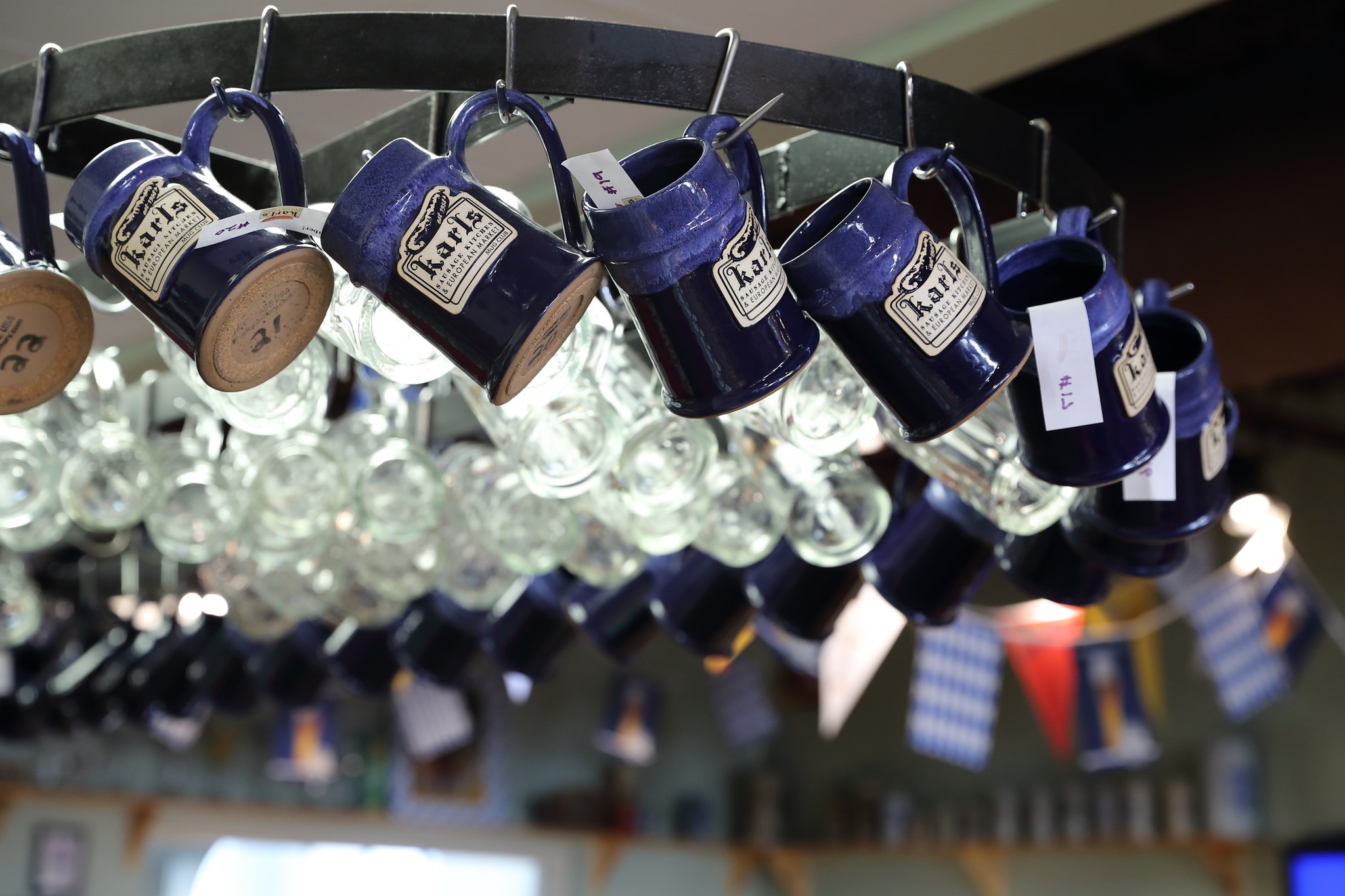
[
  {"left": 440, "top": 187, "right": 659, "bottom": 701},
  {"left": 778, "top": 146, "right": 1033, "bottom": 444},
  {"left": 0, "top": 123, "right": 94, "bottom": 417},
  {"left": 1074, "top": 277, "right": 1233, "bottom": 545},
  {"left": 63, "top": 88, "right": 335, "bottom": 393},
  {"left": 0, "top": 352, "right": 163, "bottom": 737},
  {"left": 874, "top": 389, "right": 1190, "bottom": 628},
  {"left": 132, "top": 204, "right": 440, "bottom": 722},
  {"left": 306, "top": 89, "right": 605, "bottom": 406},
  {"left": 607, "top": 309, "right": 893, "bottom": 654},
  {"left": 580, "top": 114, "right": 821, "bottom": 418},
  {"left": 993, "top": 205, "right": 1171, "bottom": 489}
]
